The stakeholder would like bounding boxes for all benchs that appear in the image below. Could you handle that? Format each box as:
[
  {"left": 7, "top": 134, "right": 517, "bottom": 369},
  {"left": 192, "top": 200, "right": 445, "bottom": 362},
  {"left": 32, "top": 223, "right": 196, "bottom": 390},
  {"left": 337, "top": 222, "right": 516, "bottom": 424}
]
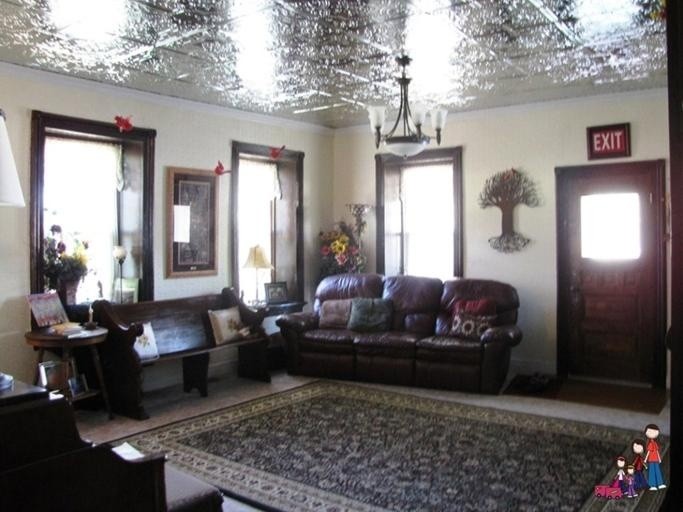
[{"left": 91, "top": 287, "right": 271, "bottom": 420}]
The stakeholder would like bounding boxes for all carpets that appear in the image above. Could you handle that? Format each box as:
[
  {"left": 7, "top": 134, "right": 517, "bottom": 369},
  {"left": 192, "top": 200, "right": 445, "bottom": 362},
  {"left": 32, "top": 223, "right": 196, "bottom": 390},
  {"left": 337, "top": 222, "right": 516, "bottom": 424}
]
[{"left": 102, "top": 375, "right": 672, "bottom": 512}]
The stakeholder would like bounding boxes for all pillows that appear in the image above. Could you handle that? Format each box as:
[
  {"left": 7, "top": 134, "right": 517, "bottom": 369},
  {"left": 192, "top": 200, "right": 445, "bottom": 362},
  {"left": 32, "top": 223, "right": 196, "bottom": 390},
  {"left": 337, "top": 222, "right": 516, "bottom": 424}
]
[
  {"left": 207, "top": 305, "right": 243, "bottom": 346},
  {"left": 119, "top": 320, "right": 159, "bottom": 360},
  {"left": 346, "top": 297, "right": 394, "bottom": 331},
  {"left": 446, "top": 297, "right": 498, "bottom": 338}
]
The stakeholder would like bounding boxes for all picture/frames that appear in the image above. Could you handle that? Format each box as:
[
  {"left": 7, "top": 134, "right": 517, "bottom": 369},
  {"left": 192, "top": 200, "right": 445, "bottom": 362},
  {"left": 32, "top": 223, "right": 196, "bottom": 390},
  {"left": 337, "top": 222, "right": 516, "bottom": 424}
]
[
  {"left": 264, "top": 281, "right": 289, "bottom": 305},
  {"left": 166, "top": 165, "right": 220, "bottom": 279}
]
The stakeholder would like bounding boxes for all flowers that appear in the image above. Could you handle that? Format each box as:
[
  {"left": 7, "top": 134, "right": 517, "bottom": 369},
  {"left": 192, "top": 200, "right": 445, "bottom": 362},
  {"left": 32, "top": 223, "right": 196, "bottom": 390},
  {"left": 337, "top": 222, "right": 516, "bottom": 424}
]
[
  {"left": 41, "top": 225, "right": 89, "bottom": 289},
  {"left": 320, "top": 220, "right": 365, "bottom": 277}
]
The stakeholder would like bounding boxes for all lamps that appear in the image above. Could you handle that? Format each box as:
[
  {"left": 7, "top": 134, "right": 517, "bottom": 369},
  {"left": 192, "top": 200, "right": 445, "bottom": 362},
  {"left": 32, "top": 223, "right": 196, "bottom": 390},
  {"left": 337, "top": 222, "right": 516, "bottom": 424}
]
[
  {"left": 114, "top": 245, "right": 127, "bottom": 303},
  {"left": 0, "top": 115, "right": 26, "bottom": 390},
  {"left": 366, "top": 46, "right": 448, "bottom": 160},
  {"left": 243, "top": 246, "right": 274, "bottom": 305}
]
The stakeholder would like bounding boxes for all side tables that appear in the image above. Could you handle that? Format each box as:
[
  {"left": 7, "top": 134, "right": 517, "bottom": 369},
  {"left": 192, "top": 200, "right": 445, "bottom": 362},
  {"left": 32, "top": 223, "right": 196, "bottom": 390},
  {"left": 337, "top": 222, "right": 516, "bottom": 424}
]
[
  {"left": 250, "top": 301, "right": 307, "bottom": 316},
  {"left": 24, "top": 328, "right": 116, "bottom": 421}
]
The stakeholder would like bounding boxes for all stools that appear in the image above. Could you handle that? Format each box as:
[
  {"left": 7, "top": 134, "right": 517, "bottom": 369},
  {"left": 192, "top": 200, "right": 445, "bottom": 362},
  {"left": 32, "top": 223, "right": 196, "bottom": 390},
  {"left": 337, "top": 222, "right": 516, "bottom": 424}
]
[{"left": 163, "top": 463, "right": 223, "bottom": 512}]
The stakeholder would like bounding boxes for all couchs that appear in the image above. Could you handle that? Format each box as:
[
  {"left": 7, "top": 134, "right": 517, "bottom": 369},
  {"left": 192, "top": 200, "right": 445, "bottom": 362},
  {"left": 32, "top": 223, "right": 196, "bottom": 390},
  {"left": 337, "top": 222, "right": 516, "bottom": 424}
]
[{"left": 276, "top": 272, "right": 523, "bottom": 396}]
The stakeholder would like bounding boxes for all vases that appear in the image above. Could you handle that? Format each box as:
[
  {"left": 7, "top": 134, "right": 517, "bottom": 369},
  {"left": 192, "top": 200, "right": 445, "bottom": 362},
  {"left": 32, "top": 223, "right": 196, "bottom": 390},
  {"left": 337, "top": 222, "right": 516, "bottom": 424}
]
[{"left": 64, "top": 279, "right": 80, "bottom": 306}]
